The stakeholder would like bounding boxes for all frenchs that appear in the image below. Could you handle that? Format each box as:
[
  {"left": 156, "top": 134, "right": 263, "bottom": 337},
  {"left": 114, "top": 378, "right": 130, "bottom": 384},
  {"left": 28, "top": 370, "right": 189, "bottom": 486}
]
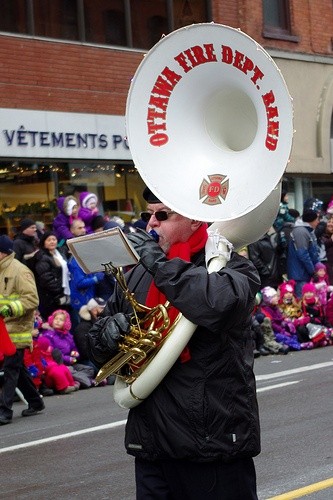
[{"left": 92, "top": 21, "right": 294, "bottom": 410}]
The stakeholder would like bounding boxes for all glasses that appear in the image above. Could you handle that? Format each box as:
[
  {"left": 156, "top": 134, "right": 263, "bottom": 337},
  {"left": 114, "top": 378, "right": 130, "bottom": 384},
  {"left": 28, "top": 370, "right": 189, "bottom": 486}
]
[{"left": 139, "top": 211, "right": 177, "bottom": 222}]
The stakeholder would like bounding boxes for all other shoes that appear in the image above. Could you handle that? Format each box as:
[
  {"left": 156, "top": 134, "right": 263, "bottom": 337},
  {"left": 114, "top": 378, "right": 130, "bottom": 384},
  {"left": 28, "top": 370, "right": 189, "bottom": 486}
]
[
  {"left": 21, "top": 404, "right": 46, "bottom": 416},
  {"left": 0, "top": 414, "right": 14, "bottom": 425},
  {"left": 252, "top": 336, "right": 333, "bottom": 359}
]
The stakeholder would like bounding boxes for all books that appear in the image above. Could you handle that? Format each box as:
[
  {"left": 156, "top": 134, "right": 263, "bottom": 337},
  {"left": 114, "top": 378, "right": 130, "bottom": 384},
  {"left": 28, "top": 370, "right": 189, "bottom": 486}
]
[{"left": 66, "top": 228, "right": 142, "bottom": 274}]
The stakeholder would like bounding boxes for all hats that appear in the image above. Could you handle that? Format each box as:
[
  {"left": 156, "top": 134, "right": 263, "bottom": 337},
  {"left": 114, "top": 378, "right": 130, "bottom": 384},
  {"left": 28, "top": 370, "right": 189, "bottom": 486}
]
[
  {"left": 78, "top": 297, "right": 107, "bottom": 321},
  {"left": 65, "top": 199, "right": 78, "bottom": 217},
  {"left": 260, "top": 286, "right": 277, "bottom": 303},
  {"left": 315, "top": 262, "right": 326, "bottom": 271},
  {"left": 301, "top": 284, "right": 316, "bottom": 295},
  {"left": 0, "top": 234, "right": 14, "bottom": 253},
  {"left": 39, "top": 230, "right": 56, "bottom": 249},
  {"left": 278, "top": 279, "right": 300, "bottom": 304},
  {"left": 18, "top": 218, "right": 35, "bottom": 232},
  {"left": 91, "top": 216, "right": 105, "bottom": 231},
  {"left": 302, "top": 209, "right": 317, "bottom": 222},
  {"left": 86, "top": 197, "right": 97, "bottom": 210},
  {"left": 288, "top": 208, "right": 299, "bottom": 218}
]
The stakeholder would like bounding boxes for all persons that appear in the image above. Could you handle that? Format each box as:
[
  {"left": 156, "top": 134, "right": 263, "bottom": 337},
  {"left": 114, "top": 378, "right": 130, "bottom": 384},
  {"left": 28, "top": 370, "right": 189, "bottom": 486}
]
[
  {"left": 246, "top": 203, "right": 333, "bottom": 293},
  {"left": 77, "top": 297, "right": 117, "bottom": 386},
  {"left": 278, "top": 283, "right": 326, "bottom": 349},
  {"left": 21, "top": 322, "right": 79, "bottom": 394},
  {"left": 297, "top": 260, "right": 333, "bottom": 349},
  {"left": 0, "top": 235, "right": 45, "bottom": 425},
  {"left": 91, "top": 185, "right": 261, "bottom": 500},
  {"left": 286, "top": 209, "right": 322, "bottom": 302},
  {"left": 79, "top": 191, "right": 99, "bottom": 235},
  {"left": 271, "top": 190, "right": 296, "bottom": 248},
  {"left": 250, "top": 291, "right": 268, "bottom": 358},
  {"left": 66, "top": 255, "right": 105, "bottom": 334},
  {"left": 31, "top": 230, "right": 72, "bottom": 316},
  {"left": 259, "top": 286, "right": 312, "bottom": 352},
  {"left": 53, "top": 195, "right": 92, "bottom": 244},
  {"left": 11, "top": 218, "right": 41, "bottom": 267},
  {"left": 42, "top": 309, "right": 95, "bottom": 387},
  {"left": 57, "top": 219, "right": 86, "bottom": 261}
]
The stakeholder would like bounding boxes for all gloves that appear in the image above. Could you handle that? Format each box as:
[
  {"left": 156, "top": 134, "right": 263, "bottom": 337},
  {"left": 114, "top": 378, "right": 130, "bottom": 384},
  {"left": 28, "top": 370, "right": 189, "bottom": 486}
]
[
  {"left": 127, "top": 225, "right": 167, "bottom": 277},
  {"left": 97, "top": 311, "right": 132, "bottom": 354},
  {"left": 312, "top": 273, "right": 318, "bottom": 282}
]
